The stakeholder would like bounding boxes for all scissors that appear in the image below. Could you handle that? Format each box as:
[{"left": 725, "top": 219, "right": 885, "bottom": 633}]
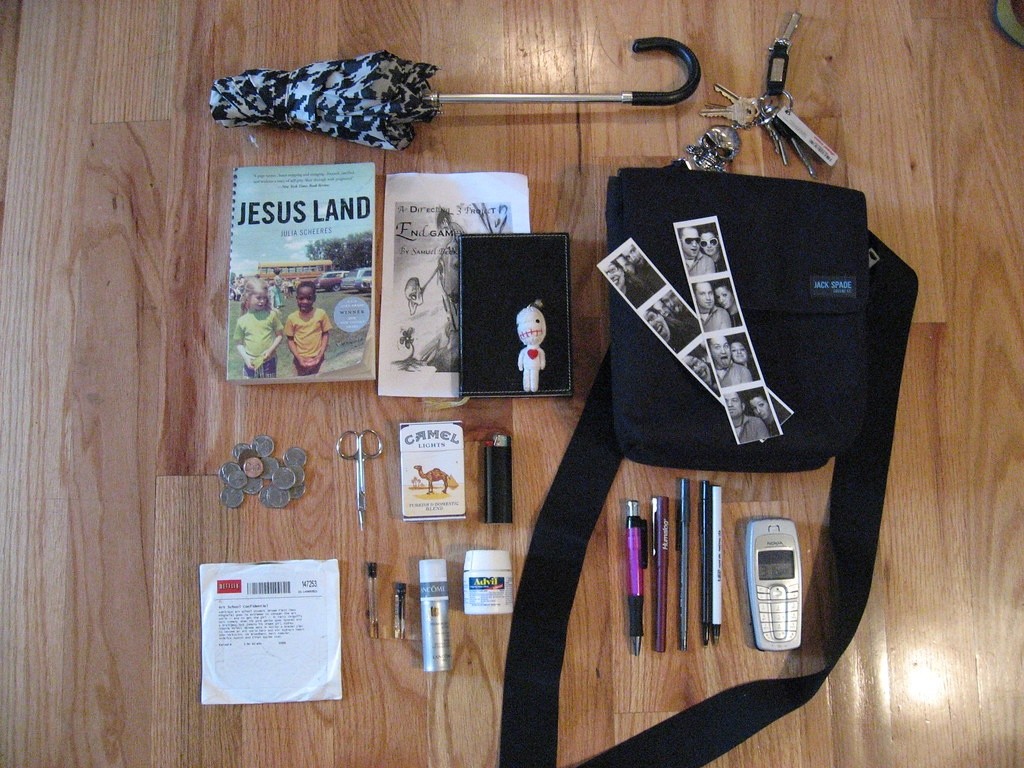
[{"left": 334, "top": 428, "right": 384, "bottom": 533}]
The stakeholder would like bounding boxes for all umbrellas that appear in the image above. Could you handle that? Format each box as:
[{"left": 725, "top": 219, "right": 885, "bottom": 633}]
[{"left": 211, "top": 36, "right": 701, "bottom": 152}]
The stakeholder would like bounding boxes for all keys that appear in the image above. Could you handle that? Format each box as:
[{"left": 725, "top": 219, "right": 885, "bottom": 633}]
[{"left": 699, "top": 12, "right": 839, "bottom": 178}]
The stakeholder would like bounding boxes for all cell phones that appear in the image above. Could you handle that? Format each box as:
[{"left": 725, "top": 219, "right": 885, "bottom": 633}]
[{"left": 747, "top": 517, "right": 803, "bottom": 652}]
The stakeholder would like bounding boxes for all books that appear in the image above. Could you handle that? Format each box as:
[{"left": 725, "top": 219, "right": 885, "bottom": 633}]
[
  {"left": 459, "top": 233, "right": 573, "bottom": 397},
  {"left": 378, "top": 173, "right": 530, "bottom": 397},
  {"left": 226, "top": 162, "right": 376, "bottom": 384}
]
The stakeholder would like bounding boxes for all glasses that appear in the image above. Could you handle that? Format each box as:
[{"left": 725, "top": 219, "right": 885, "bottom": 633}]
[
  {"left": 646, "top": 311, "right": 658, "bottom": 323},
  {"left": 606, "top": 267, "right": 617, "bottom": 274},
  {"left": 679, "top": 238, "right": 701, "bottom": 245},
  {"left": 698, "top": 238, "right": 718, "bottom": 248},
  {"left": 689, "top": 356, "right": 701, "bottom": 370}
]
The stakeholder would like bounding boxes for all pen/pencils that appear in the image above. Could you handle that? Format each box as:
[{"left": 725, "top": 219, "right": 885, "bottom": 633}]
[
  {"left": 621, "top": 498, "right": 649, "bottom": 655},
  {"left": 674, "top": 476, "right": 690, "bottom": 651},
  {"left": 700, "top": 480, "right": 725, "bottom": 648},
  {"left": 651, "top": 495, "right": 671, "bottom": 653}
]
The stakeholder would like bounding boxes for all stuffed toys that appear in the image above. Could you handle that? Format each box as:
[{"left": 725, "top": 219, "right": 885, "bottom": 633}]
[{"left": 516, "top": 305, "right": 547, "bottom": 392}]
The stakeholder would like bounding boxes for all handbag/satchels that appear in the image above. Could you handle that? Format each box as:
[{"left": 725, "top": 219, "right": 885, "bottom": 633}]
[{"left": 605, "top": 166, "right": 869, "bottom": 472}]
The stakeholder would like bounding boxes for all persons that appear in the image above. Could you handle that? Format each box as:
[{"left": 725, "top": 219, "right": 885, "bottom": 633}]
[
  {"left": 604, "top": 244, "right": 665, "bottom": 309},
  {"left": 683, "top": 355, "right": 720, "bottom": 397},
  {"left": 232, "top": 268, "right": 301, "bottom": 308},
  {"left": 694, "top": 282, "right": 742, "bottom": 332},
  {"left": 644, "top": 291, "right": 700, "bottom": 352},
  {"left": 725, "top": 392, "right": 779, "bottom": 443},
  {"left": 680, "top": 227, "right": 727, "bottom": 276},
  {"left": 709, "top": 336, "right": 760, "bottom": 388},
  {"left": 234, "top": 279, "right": 284, "bottom": 378},
  {"left": 284, "top": 280, "right": 330, "bottom": 376}
]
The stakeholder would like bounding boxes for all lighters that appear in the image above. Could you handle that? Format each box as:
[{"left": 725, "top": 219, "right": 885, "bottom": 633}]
[{"left": 484, "top": 434, "right": 512, "bottom": 523}]
